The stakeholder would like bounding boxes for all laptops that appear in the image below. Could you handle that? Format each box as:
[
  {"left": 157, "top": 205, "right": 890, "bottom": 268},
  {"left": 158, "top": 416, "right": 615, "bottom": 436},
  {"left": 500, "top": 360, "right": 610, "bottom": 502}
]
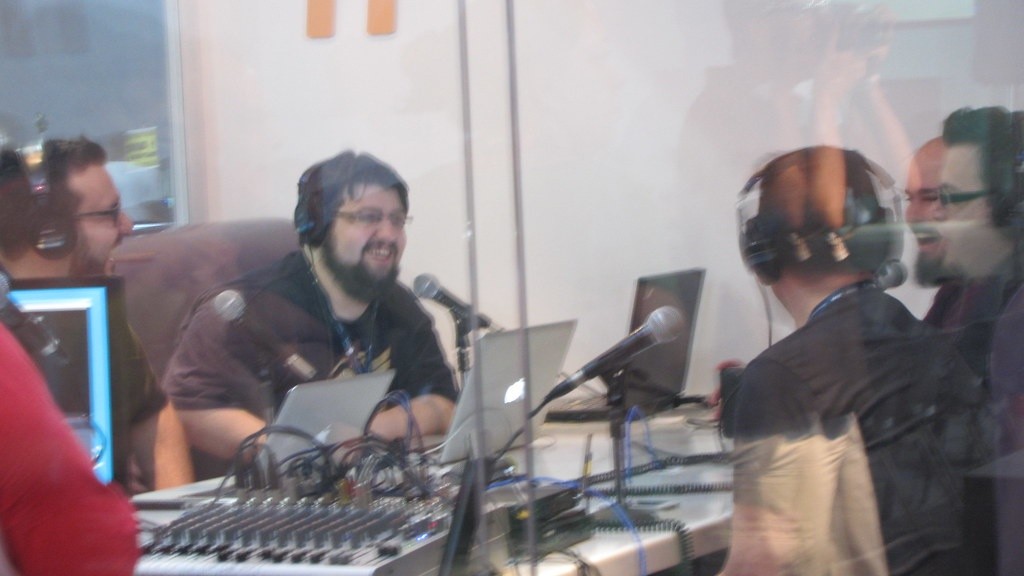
[
  {"left": 547, "top": 268, "right": 706, "bottom": 424},
  {"left": 389, "top": 318, "right": 576, "bottom": 466},
  {"left": 132, "top": 367, "right": 395, "bottom": 511}
]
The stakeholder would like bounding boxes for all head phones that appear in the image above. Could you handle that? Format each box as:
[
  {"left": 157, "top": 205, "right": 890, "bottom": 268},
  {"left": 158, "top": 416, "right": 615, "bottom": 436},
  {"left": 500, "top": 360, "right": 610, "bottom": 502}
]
[
  {"left": 997, "top": 110, "right": 1024, "bottom": 228},
  {"left": 295, "top": 160, "right": 344, "bottom": 246},
  {"left": 738, "top": 152, "right": 905, "bottom": 287},
  {"left": 15, "top": 142, "right": 77, "bottom": 260}
]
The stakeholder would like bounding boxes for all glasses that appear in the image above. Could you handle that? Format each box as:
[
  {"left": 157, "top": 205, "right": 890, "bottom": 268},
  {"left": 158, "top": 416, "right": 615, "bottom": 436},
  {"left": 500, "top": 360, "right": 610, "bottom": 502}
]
[
  {"left": 74, "top": 195, "right": 123, "bottom": 227},
  {"left": 333, "top": 206, "right": 413, "bottom": 234},
  {"left": 929, "top": 186, "right": 993, "bottom": 218}
]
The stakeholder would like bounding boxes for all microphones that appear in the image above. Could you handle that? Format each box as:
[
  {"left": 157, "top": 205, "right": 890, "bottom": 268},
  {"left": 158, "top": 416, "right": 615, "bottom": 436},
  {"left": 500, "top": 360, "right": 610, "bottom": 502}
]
[
  {"left": 547, "top": 306, "right": 687, "bottom": 403},
  {"left": 875, "top": 260, "right": 909, "bottom": 291},
  {"left": 0, "top": 273, "right": 68, "bottom": 366},
  {"left": 411, "top": 274, "right": 503, "bottom": 335},
  {"left": 216, "top": 290, "right": 318, "bottom": 382}
]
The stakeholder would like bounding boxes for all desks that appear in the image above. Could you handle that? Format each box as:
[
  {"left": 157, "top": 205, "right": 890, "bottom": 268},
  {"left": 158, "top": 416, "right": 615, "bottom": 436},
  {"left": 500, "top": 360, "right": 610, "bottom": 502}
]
[{"left": 126, "top": 412, "right": 734, "bottom": 576}]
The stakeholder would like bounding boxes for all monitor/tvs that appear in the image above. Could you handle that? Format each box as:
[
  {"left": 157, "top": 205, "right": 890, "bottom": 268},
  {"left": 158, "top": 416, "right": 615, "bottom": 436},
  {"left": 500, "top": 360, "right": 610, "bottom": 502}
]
[{"left": 7, "top": 275, "right": 129, "bottom": 496}]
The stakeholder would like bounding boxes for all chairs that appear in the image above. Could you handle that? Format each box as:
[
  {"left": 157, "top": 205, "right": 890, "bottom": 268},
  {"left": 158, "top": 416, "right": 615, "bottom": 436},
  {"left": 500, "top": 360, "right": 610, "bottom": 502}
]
[{"left": 103, "top": 216, "right": 300, "bottom": 493}]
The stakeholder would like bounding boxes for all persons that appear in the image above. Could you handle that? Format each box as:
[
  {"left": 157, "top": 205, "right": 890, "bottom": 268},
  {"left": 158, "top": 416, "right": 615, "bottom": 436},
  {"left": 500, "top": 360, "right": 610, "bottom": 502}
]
[
  {"left": 718, "top": 145, "right": 992, "bottom": 576},
  {"left": 163, "top": 150, "right": 459, "bottom": 480},
  {"left": 0, "top": 138, "right": 193, "bottom": 494},
  {"left": 0, "top": 323, "right": 136, "bottom": 576},
  {"left": 905, "top": 107, "right": 1023, "bottom": 576}
]
